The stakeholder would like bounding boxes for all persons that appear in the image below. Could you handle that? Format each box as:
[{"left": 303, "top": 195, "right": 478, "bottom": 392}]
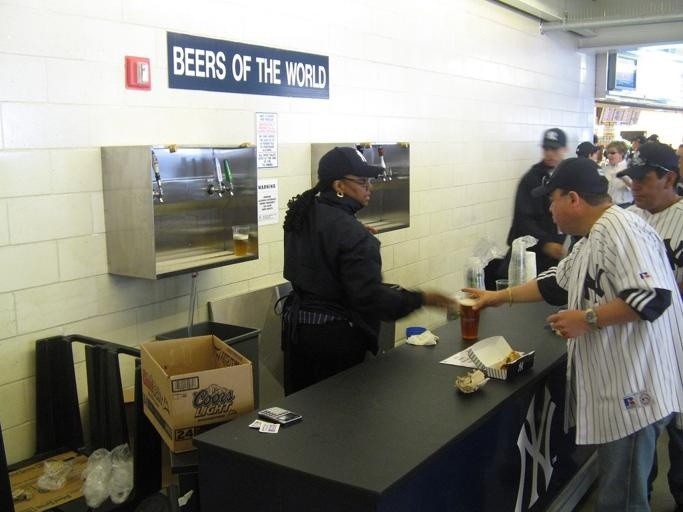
[
  {"left": 460, "top": 156, "right": 683, "bottom": 512},
  {"left": 281, "top": 147, "right": 459, "bottom": 397},
  {"left": 496, "top": 125, "right": 682, "bottom": 277},
  {"left": 615, "top": 141, "right": 682, "bottom": 512}
]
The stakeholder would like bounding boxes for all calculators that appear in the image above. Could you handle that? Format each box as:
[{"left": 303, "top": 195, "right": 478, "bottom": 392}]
[{"left": 258, "top": 407, "right": 302, "bottom": 426}]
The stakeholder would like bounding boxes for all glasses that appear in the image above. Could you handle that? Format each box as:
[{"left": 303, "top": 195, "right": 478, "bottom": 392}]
[{"left": 343, "top": 176, "right": 368, "bottom": 187}]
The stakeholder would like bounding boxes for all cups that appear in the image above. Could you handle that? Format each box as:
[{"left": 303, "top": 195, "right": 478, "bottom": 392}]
[
  {"left": 506, "top": 240, "right": 538, "bottom": 290},
  {"left": 231, "top": 224, "right": 250, "bottom": 257},
  {"left": 494, "top": 278, "right": 509, "bottom": 291},
  {"left": 458, "top": 292, "right": 481, "bottom": 340}
]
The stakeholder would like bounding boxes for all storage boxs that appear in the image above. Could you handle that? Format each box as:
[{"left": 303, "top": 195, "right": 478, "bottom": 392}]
[{"left": 140, "top": 334, "right": 254, "bottom": 453}]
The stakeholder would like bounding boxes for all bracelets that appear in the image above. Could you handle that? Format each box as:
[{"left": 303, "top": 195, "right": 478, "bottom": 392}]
[{"left": 507, "top": 288, "right": 512, "bottom": 308}]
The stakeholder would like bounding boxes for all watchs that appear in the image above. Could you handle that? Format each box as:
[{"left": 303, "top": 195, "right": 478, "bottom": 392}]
[{"left": 584, "top": 308, "right": 601, "bottom": 332}]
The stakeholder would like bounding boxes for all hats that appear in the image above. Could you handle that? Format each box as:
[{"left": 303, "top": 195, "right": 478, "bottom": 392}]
[
  {"left": 530, "top": 157, "right": 609, "bottom": 199},
  {"left": 316, "top": 145, "right": 384, "bottom": 191},
  {"left": 576, "top": 141, "right": 603, "bottom": 158},
  {"left": 615, "top": 142, "right": 680, "bottom": 180},
  {"left": 540, "top": 128, "right": 566, "bottom": 150}
]
